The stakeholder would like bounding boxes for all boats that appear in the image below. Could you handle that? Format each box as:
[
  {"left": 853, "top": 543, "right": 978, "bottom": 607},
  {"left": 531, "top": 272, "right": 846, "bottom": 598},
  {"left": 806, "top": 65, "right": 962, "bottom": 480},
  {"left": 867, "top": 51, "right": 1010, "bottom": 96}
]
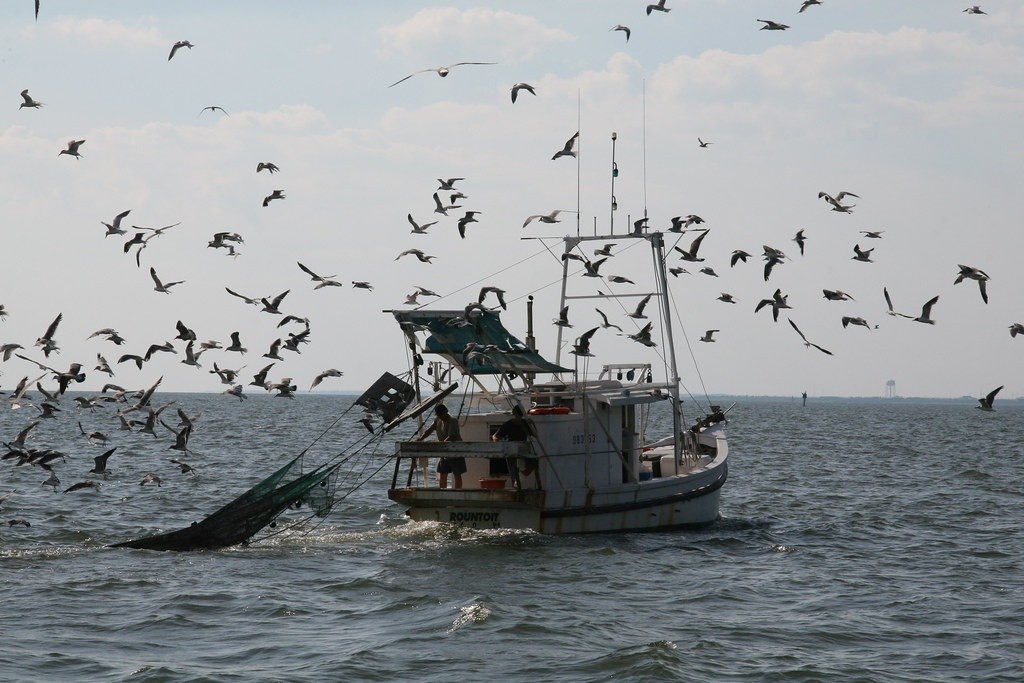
[{"left": 381, "top": 75, "right": 737, "bottom": 538}]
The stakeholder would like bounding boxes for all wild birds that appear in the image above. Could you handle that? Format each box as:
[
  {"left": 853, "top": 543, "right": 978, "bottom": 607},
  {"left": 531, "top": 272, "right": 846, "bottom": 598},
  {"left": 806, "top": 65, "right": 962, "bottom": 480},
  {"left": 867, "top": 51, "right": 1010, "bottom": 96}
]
[{"left": 0, "top": 0, "right": 1024, "bottom": 528}]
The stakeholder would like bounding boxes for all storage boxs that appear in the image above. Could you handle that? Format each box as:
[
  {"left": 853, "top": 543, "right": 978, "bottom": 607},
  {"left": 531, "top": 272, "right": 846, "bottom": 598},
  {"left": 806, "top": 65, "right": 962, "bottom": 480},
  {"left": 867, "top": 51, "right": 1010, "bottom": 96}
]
[
  {"left": 660, "top": 455, "right": 711, "bottom": 477},
  {"left": 642, "top": 450, "right": 688, "bottom": 478},
  {"left": 654, "top": 445, "right": 675, "bottom": 450},
  {"left": 639, "top": 461, "right": 652, "bottom": 482}
]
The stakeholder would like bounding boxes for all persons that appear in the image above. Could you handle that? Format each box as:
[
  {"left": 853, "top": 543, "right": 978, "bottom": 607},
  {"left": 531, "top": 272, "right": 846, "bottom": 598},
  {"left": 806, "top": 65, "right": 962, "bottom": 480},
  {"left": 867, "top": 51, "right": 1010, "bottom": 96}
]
[
  {"left": 417, "top": 403, "right": 467, "bottom": 488},
  {"left": 492, "top": 405, "right": 536, "bottom": 487}
]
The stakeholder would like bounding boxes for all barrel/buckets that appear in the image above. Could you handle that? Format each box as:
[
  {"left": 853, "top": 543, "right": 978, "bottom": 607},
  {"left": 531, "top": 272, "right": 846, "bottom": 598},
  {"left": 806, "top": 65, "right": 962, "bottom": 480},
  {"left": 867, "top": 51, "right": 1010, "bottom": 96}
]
[{"left": 478, "top": 478, "right": 506, "bottom": 488}]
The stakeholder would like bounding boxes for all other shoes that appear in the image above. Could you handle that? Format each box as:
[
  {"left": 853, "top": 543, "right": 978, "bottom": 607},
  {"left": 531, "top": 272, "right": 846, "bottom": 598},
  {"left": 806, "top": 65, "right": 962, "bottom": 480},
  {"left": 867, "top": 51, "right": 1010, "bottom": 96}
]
[{"left": 521, "top": 471, "right": 530, "bottom": 476}]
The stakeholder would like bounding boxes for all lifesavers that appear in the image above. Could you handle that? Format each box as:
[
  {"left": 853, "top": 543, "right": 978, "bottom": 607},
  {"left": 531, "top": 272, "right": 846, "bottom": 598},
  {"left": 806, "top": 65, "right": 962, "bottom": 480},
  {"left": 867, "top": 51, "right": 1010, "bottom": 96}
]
[{"left": 528, "top": 407, "right": 572, "bottom": 416}]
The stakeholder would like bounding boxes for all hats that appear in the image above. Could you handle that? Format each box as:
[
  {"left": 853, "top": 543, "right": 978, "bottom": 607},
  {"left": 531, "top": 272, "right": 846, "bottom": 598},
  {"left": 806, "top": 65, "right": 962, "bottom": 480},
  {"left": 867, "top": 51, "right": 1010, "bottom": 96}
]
[{"left": 435, "top": 404, "right": 447, "bottom": 415}]
